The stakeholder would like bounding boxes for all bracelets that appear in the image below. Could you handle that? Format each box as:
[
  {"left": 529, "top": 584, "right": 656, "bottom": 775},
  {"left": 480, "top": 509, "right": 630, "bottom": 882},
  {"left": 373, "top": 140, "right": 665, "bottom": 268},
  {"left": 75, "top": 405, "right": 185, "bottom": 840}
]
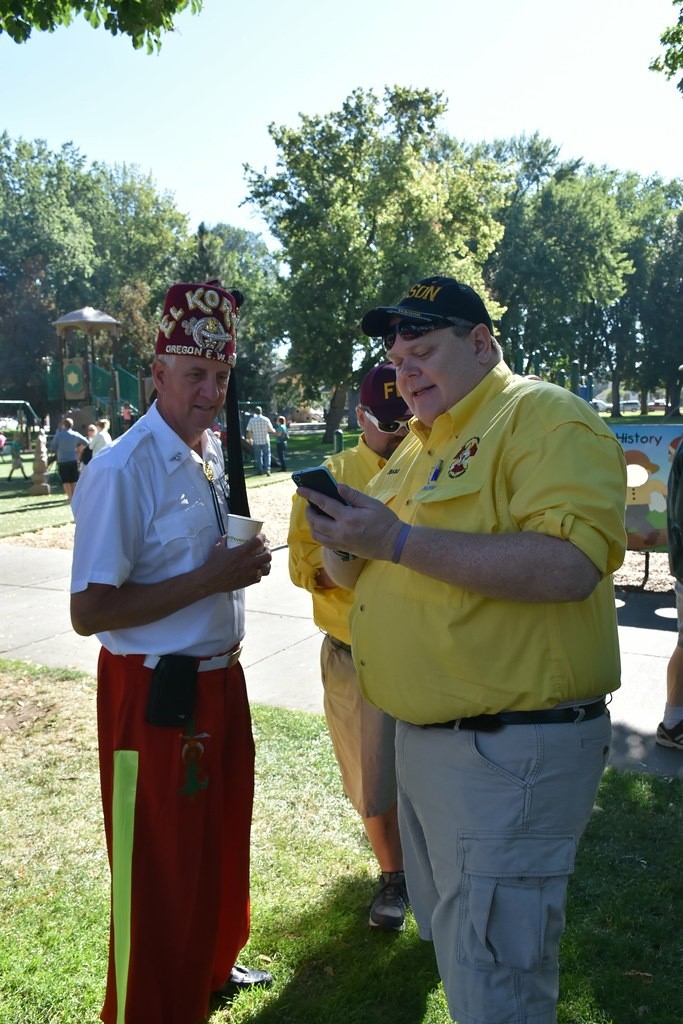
[
  {"left": 392, "top": 524, "right": 411, "bottom": 564},
  {"left": 334, "top": 551, "right": 356, "bottom": 562}
]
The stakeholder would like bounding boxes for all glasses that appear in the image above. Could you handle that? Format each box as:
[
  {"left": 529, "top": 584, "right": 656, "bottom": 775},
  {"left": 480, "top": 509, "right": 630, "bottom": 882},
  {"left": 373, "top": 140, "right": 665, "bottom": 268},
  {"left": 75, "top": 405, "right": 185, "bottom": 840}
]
[
  {"left": 381, "top": 317, "right": 455, "bottom": 351},
  {"left": 362, "top": 408, "right": 411, "bottom": 434}
]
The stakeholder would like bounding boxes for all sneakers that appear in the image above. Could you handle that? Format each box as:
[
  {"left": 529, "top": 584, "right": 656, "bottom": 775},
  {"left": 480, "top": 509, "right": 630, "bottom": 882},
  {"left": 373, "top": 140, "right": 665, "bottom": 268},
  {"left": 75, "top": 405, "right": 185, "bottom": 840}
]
[
  {"left": 655, "top": 719, "right": 683, "bottom": 750},
  {"left": 368, "top": 875, "right": 409, "bottom": 931}
]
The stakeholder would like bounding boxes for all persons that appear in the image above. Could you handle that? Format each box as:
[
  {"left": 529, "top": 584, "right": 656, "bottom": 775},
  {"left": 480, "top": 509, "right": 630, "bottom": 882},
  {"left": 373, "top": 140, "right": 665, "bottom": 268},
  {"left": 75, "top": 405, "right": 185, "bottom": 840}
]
[
  {"left": 289, "top": 361, "right": 408, "bottom": 931},
  {"left": 7, "top": 433, "right": 31, "bottom": 481},
  {"left": 275, "top": 416, "right": 288, "bottom": 472},
  {"left": 657, "top": 440, "right": 683, "bottom": 750},
  {"left": 296, "top": 276, "right": 627, "bottom": 1024},
  {"left": 48, "top": 418, "right": 89, "bottom": 503},
  {"left": 0, "top": 431, "right": 7, "bottom": 463},
  {"left": 246, "top": 407, "right": 275, "bottom": 476},
  {"left": 114, "top": 403, "right": 137, "bottom": 438},
  {"left": 81, "top": 419, "right": 111, "bottom": 470},
  {"left": 69, "top": 282, "right": 273, "bottom": 1024},
  {"left": 213, "top": 431, "right": 222, "bottom": 448}
]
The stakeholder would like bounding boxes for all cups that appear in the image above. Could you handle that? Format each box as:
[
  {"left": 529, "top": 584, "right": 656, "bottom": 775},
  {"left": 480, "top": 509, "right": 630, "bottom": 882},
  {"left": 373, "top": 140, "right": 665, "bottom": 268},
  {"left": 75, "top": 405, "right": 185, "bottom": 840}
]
[{"left": 227, "top": 514, "right": 265, "bottom": 549}]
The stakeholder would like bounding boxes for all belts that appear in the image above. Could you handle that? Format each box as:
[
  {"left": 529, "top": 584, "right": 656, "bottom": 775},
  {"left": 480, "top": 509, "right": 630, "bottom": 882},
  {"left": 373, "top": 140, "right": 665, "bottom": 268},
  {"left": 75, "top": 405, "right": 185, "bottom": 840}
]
[
  {"left": 328, "top": 631, "right": 351, "bottom": 654},
  {"left": 143, "top": 643, "right": 244, "bottom": 672},
  {"left": 427, "top": 697, "right": 606, "bottom": 731}
]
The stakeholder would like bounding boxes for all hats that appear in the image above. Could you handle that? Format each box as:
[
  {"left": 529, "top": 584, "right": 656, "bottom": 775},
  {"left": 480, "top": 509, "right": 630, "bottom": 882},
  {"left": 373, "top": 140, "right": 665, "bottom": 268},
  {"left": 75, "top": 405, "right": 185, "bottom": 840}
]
[
  {"left": 155, "top": 283, "right": 237, "bottom": 368},
  {"left": 361, "top": 276, "right": 494, "bottom": 345},
  {"left": 359, "top": 362, "right": 414, "bottom": 423}
]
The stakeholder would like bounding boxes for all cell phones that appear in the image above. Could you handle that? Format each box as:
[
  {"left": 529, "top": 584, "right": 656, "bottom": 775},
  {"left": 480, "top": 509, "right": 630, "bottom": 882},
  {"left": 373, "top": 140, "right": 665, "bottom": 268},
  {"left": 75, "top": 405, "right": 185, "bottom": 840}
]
[{"left": 291, "top": 466, "right": 353, "bottom": 519}]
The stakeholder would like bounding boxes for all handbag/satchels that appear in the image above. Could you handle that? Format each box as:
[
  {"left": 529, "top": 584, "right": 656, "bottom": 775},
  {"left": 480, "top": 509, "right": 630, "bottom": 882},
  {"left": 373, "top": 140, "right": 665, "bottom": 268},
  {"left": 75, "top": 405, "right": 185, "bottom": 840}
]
[{"left": 278, "top": 433, "right": 290, "bottom": 441}]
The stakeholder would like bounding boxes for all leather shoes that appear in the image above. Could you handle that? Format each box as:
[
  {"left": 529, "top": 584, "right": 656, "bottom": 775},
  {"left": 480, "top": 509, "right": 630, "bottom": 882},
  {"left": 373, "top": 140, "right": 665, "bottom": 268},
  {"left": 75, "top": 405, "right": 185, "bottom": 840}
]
[{"left": 219, "top": 964, "right": 272, "bottom": 998}]
